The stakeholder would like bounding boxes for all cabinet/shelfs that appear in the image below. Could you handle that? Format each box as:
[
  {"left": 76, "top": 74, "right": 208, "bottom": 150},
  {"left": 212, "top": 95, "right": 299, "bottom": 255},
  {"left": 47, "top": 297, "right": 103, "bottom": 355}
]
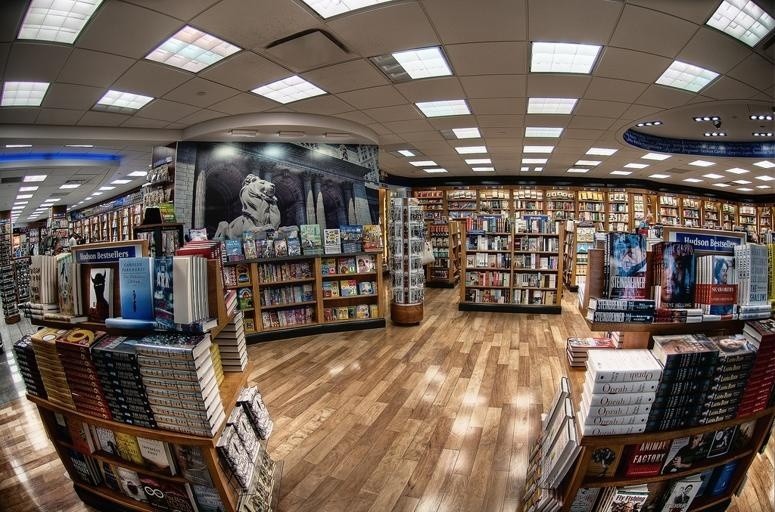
[
  {"left": 426, "top": 222, "right": 459, "bottom": 285},
  {"left": 23, "top": 258, "right": 285, "bottom": 512},
  {"left": 223, "top": 248, "right": 385, "bottom": 337},
  {"left": 460, "top": 219, "right": 565, "bottom": 311},
  {"left": 550, "top": 249, "right": 774, "bottom": 512},
  {"left": 390, "top": 196, "right": 425, "bottom": 322},
  {"left": 566, "top": 222, "right": 597, "bottom": 291},
  {"left": 412, "top": 187, "right": 774, "bottom": 274},
  {"left": 0, "top": 173, "right": 178, "bottom": 322}
]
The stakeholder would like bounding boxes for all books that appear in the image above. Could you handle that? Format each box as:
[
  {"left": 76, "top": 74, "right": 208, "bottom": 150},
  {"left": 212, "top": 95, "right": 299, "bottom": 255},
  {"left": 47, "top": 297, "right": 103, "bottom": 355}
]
[
  {"left": 385, "top": 188, "right": 774, "bottom": 370},
  {"left": 0, "top": 186, "right": 383, "bottom": 335},
  {"left": 13, "top": 311, "right": 276, "bottom": 512},
  {"left": 522, "top": 318, "right": 774, "bottom": 512}
]
